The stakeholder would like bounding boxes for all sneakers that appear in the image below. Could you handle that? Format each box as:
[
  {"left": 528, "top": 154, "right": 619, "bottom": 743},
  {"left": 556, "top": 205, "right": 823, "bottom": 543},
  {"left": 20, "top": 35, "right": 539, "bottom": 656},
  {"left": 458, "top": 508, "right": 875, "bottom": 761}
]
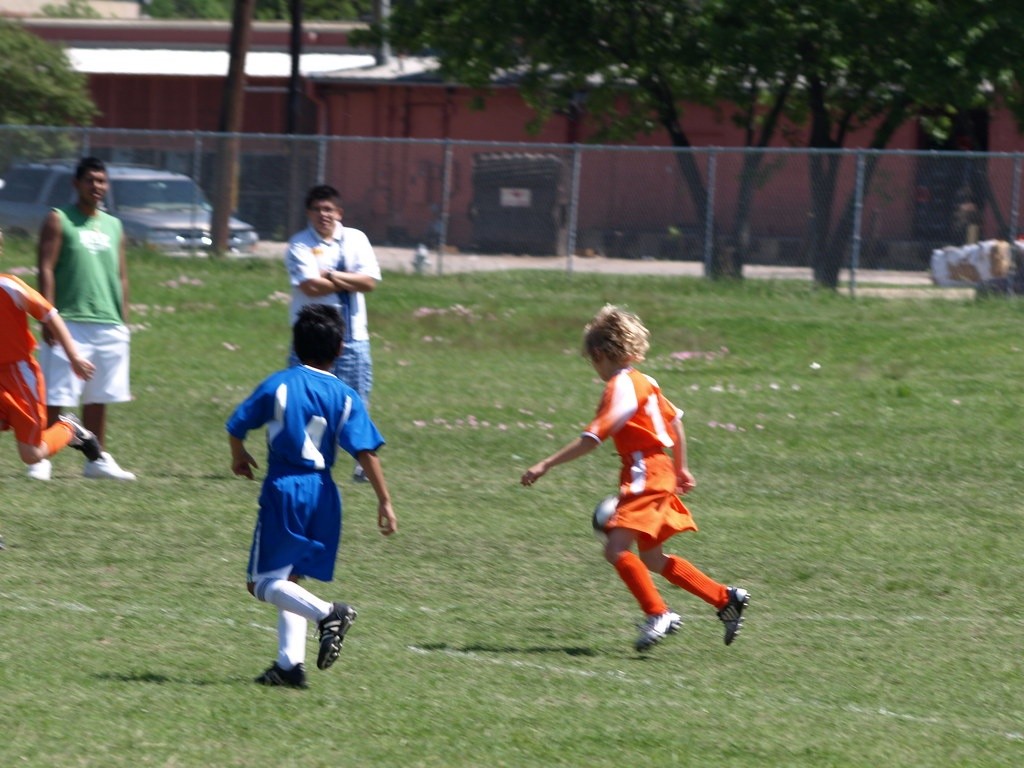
[
  {"left": 85, "top": 451, "right": 136, "bottom": 481},
  {"left": 314, "top": 600, "right": 357, "bottom": 670},
  {"left": 27, "top": 456, "right": 52, "bottom": 484},
  {"left": 635, "top": 610, "right": 683, "bottom": 649},
  {"left": 59, "top": 412, "right": 100, "bottom": 461},
  {"left": 256, "top": 661, "right": 308, "bottom": 688},
  {"left": 717, "top": 587, "right": 751, "bottom": 646}
]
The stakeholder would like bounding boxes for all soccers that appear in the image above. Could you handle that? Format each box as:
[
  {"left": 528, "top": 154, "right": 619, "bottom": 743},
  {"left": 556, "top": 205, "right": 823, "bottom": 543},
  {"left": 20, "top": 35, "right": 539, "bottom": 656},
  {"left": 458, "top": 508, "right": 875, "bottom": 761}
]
[{"left": 592, "top": 496, "right": 620, "bottom": 549}]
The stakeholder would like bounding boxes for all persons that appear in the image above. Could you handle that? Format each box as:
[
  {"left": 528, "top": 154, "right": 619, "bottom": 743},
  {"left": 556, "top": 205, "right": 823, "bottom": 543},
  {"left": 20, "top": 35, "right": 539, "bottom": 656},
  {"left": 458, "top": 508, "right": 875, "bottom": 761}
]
[
  {"left": 224, "top": 182, "right": 400, "bottom": 689},
  {"left": 519, "top": 302, "right": 750, "bottom": 653},
  {"left": 0, "top": 156, "right": 138, "bottom": 487}
]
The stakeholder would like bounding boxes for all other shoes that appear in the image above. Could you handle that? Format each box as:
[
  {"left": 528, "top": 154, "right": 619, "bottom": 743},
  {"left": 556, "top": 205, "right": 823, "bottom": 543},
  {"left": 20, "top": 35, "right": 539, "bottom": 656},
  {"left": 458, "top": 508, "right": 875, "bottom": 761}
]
[{"left": 355, "top": 466, "right": 371, "bottom": 483}]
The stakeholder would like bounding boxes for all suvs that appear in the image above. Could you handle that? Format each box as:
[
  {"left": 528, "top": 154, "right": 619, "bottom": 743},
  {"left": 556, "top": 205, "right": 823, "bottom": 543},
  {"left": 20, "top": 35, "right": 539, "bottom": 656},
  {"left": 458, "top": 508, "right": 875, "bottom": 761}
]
[{"left": 0, "top": 158, "right": 260, "bottom": 260}]
